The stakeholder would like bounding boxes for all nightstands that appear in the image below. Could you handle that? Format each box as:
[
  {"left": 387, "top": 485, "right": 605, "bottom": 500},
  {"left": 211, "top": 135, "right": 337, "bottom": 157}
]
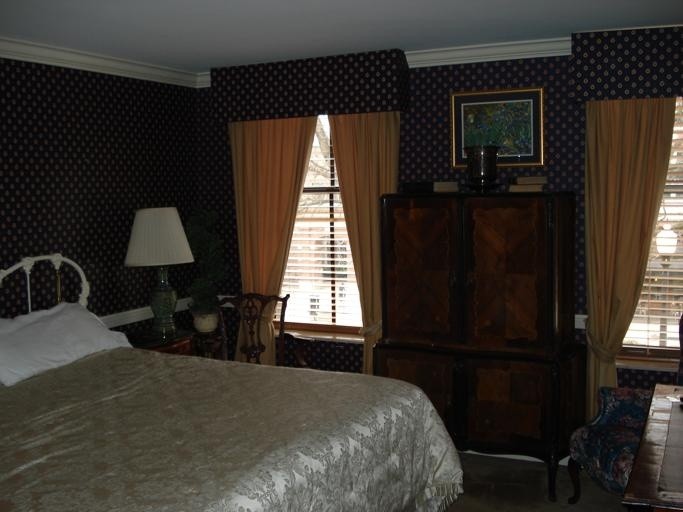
[{"left": 128, "top": 329, "right": 195, "bottom": 356}]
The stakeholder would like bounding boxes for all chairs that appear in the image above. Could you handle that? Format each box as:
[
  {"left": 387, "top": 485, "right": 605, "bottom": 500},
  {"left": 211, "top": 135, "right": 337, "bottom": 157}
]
[
  {"left": 214, "top": 293, "right": 289, "bottom": 366},
  {"left": 568, "top": 386, "right": 653, "bottom": 503}
]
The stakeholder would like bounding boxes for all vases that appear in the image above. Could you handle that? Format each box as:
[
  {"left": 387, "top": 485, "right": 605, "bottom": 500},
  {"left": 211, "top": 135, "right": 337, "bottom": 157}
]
[{"left": 464, "top": 146, "right": 501, "bottom": 192}]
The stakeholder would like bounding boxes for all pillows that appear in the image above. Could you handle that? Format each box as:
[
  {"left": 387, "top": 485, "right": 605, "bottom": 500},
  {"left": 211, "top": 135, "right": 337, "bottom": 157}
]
[{"left": 0, "top": 301, "right": 134, "bottom": 387}]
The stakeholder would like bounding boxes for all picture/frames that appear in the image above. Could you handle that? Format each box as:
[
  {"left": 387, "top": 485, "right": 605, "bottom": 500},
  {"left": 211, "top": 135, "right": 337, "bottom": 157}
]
[{"left": 451, "top": 88, "right": 544, "bottom": 168}]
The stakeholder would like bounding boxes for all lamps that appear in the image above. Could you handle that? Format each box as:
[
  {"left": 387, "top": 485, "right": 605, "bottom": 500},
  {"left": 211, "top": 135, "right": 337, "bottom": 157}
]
[{"left": 124, "top": 207, "right": 195, "bottom": 344}]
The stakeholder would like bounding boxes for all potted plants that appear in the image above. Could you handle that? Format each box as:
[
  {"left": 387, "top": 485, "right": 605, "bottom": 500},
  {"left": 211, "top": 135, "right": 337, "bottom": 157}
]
[{"left": 187, "top": 268, "right": 224, "bottom": 332}]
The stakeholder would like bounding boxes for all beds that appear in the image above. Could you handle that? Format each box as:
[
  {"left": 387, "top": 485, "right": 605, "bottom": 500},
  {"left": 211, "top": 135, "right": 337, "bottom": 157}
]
[{"left": 0, "top": 253, "right": 464, "bottom": 512}]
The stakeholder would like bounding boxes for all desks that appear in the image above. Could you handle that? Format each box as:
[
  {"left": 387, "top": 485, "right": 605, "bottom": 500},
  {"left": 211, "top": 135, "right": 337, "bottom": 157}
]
[{"left": 621, "top": 384, "right": 683, "bottom": 512}]
